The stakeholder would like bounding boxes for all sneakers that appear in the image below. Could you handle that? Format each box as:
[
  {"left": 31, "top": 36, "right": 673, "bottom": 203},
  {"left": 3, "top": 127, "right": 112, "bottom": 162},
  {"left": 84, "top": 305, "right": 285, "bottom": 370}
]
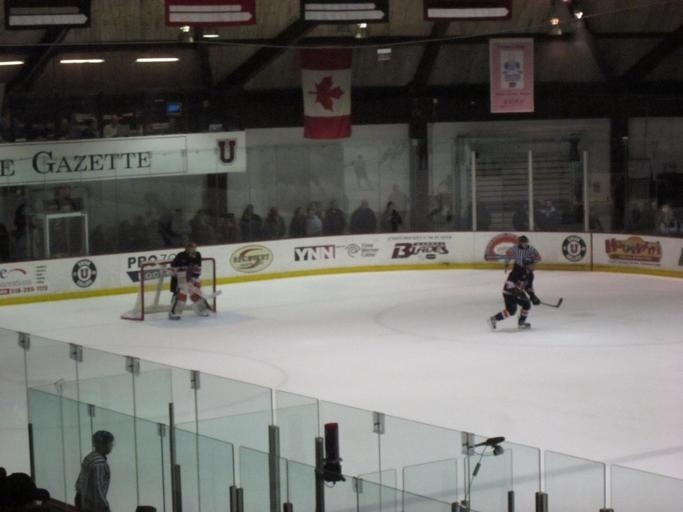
[
  {"left": 520, "top": 320, "right": 530, "bottom": 326},
  {"left": 491, "top": 316, "right": 497, "bottom": 328}
]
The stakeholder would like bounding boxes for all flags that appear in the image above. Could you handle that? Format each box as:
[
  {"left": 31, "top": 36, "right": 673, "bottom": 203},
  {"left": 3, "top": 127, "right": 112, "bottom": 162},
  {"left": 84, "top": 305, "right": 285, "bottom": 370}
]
[
  {"left": 488, "top": 35, "right": 536, "bottom": 114},
  {"left": 298, "top": 46, "right": 353, "bottom": 139}
]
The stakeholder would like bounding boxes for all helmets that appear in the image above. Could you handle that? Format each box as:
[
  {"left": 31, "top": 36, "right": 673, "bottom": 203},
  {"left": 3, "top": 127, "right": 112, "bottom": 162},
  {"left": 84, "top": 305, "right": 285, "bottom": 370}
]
[
  {"left": 520, "top": 236, "right": 528, "bottom": 242},
  {"left": 523, "top": 258, "right": 534, "bottom": 265},
  {"left": 185, "top": 242, "right": 197, "bottom": 258}
]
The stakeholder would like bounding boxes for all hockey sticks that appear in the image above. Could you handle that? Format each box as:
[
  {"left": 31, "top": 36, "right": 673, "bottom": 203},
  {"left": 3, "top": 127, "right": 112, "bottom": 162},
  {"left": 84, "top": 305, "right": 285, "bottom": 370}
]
[
  {"left": 503, "top": 290, "right": 563, "bottom": 308},
  {"left": 185, "top": 281, "right": 222, "bottom": 300}
]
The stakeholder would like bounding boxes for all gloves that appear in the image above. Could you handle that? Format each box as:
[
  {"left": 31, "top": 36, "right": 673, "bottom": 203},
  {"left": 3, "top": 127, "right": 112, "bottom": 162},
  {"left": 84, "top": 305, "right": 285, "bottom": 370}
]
[
  {"left": 512, "top": 287, "right": 522, "bottom": 302},
  {"left": 529, "top": 293, "right": 540, "bottom": 305}
]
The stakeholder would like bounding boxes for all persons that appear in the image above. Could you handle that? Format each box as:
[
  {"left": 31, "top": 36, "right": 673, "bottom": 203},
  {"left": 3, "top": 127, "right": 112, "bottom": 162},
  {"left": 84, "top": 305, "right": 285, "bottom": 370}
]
[
  {"left": 78, "top": 116, "right": 98, "bottom": 138},
  {"left": 537, "top": 201, "right": 557, "bottom": 232},
  {"left": 72, "top": 428, "right": 115, "bottom": 512},
  {"left": 484, "top": 256, "right": 539, "bottom": 332},
  {"left": 189, "top": 200, "right": 345, "bottom": 248},
  {"left": 2, "top": 200, "right": 183, "bottom": 262},
  {"left": 351, "top": 200, "right": 401, "bottom": 235},
  {"left": 101, "top": 114, "right": 120, "bottom": 138},
  {"left": 0, "top": 470, "right": 50, "bottom": 511},
  {"left": 164, "top": 241, "right": 211, "bottom": 322},
  {"left": 0, "top": 110, "right": 16, "bottom": 144},
  {"left": 503, "top": 235, "right": 540, "bottom": 272},
  {"left": 509, "top": 199, "right": 528, "bottom": 231},
  {"left": 55, "top": 118, "right": 70, "bottom": 139},
  {"left": 621, "top": 196, "right": 682, "bottom": 237}
]
